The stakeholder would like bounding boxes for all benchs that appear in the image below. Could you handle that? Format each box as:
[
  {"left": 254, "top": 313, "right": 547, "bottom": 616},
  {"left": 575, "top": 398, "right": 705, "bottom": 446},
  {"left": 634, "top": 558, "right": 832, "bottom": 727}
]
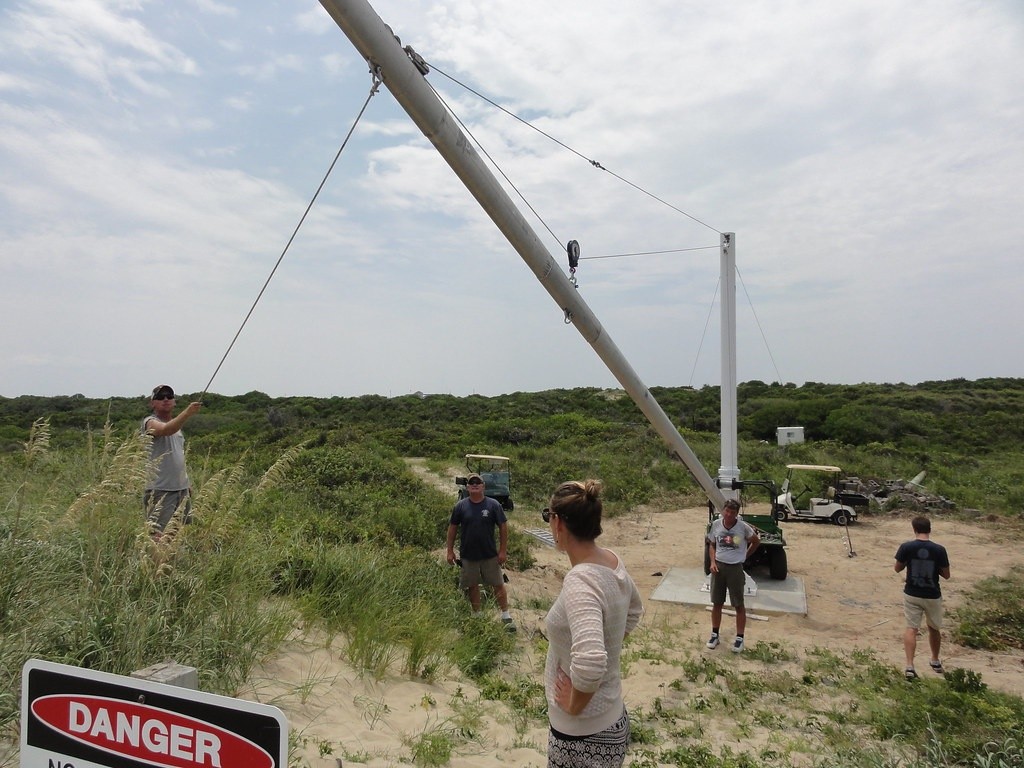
[{"left": 810, "top": 486, "right": 836, "bottom": 506}]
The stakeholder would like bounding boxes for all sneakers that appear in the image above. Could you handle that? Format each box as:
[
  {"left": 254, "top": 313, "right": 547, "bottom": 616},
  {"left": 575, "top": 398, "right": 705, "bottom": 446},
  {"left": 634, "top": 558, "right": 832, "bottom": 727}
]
[
  {"left": 706, "top": 633, "right": 720, "bottom": 649},
  {"left": 905, "top": 666, "right": 918, "bottom": 678},
  {"left": 731, "top": 636, "right": 744, "bottom": 653},
  {"left": 929, "top": 659, "right": 943, "bottom": 672}
]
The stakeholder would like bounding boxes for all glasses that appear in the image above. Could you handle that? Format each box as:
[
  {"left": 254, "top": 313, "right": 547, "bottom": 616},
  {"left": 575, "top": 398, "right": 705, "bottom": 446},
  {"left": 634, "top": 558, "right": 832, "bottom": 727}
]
[
  {"left": 728, "top": 499, "right": 738, "bottom": 508},
  {"left": 542, "top": 509, "right": 567, "bottom": 522},
  {"left": 468, "top": 480, "right": 481, "bottom": 485},
  {"left": 153, "top": 393, "right": 174, "bottom": 400}
]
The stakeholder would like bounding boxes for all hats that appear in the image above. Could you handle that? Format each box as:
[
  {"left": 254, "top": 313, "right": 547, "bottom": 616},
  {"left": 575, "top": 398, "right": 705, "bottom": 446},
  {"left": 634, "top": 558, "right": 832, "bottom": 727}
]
[
  {"left": 467, "top": 473, "right": 483, "bottom": 484},
  {"left": 151, "top": 385, "right": 174, "bottom": 399}
]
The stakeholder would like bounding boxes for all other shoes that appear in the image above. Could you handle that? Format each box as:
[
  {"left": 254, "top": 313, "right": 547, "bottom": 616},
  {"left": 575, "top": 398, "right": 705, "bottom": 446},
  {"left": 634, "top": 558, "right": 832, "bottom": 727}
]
[{"left": 501, "top": 618, "right": 516, "bottom": 632}]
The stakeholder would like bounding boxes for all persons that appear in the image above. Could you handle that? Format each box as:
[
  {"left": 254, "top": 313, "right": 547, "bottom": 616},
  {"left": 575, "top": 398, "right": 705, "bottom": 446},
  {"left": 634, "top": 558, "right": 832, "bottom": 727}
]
[
  {"left": 446, "top": 473, "right": 517, "bottom": 632},
  {"left": 542, "top": 481, "right": 645, "bottom": 768},
  {"left": 894, "top": 517, "right": 951, "bottom": 678},
  {"left": 706, "top": 499, "right": 761, "bottom": 654},
  {"left": 142, "top": 384, "right": 203, "bottom": 546}
]
